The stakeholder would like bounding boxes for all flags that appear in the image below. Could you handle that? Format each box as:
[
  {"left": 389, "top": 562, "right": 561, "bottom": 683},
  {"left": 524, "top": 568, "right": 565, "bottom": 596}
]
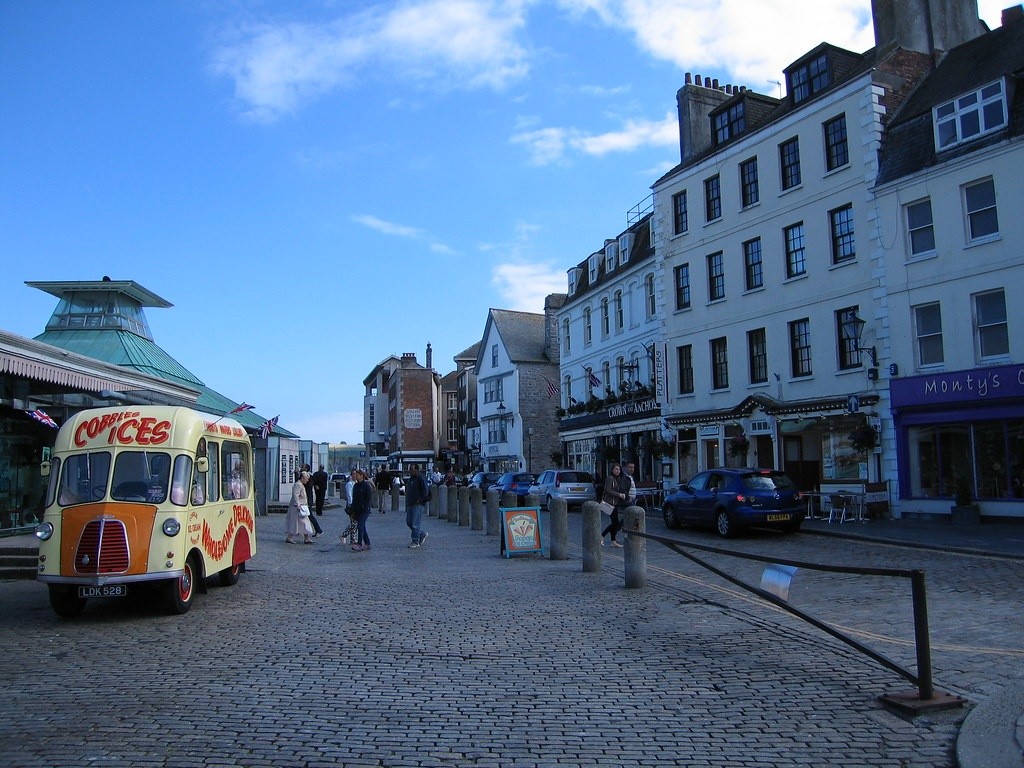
[
  {"left": 590, "top": 375, "right": 600, "bottom": 387},
  {"left": 229, "top": 404, "right": 254, "bottom": 415},
  {"left": 260, "top": 416, "right": 278, "bottom": 439},
  {"left": 26, "top": 409, "right": 59, "bottom": 429},
  {"left": 546, "top": 383, "right": 560, "bottom": 399}
]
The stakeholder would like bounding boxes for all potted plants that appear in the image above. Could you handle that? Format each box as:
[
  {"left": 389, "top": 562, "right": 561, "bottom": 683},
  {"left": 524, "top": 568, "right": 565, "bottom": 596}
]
[{"left": 951, "top": 478, "right": 981, "bottom": 530}]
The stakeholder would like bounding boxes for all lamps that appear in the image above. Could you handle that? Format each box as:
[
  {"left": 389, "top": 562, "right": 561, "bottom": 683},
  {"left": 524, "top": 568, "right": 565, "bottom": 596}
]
[
  {"left": 571, "top": 398, "right": 577, "bottom": 403},
  {"left": 591, "top": 395, "right": 599, "bottom": 400},
  {"left": 635, "top": 443, "right": 644, "bottom": 456},
  {"left": 449, "top": 409, "right": 457, "bottom": 421},
  {"left": 840, "top": 307, "right": 880, "bottom": 367},
  {"left": 496, "top": 402, "right": 514, "bottom": 428},
  {"left": 619, "top": 386, "right": 632, "bottom": 400},
  {"left": 635, "top": 381, "right": 653, "bottom": 397},
  {"left": 606, "top": 388, "right": 613, "bottom": 396}
]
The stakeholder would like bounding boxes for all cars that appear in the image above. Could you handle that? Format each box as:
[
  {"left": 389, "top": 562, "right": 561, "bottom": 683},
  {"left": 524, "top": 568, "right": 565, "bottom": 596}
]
[
  {"left": 485, "top": 471, "right": 539, "bottom": 505},
  {"left": 468, "top": 471, "right": 505, "bottom": 500},
  {"left": 523, "top": 468, "right": 597, "bottom": 512},
  {"left": 329, "top": 472, "right": 347, "bottom": 489},
  {"left": 660, "top": 466, "right": 806, "bottom": 540}
]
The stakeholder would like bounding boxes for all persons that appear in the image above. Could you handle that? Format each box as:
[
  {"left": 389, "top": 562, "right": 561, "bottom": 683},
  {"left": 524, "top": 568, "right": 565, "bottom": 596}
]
[
  {"left": 618, "top": 461, "right": 638, "bottom": 531},
  {"left": 374, "top": 464, "right": 391, "bottom": 513},
  {"left": 405, "top": 464, "right": 428, "bottom": 548},
  {"left": 339, "top": 469, "right": 375, "bottom": 550},
  {"left": 393, "top": 474, "right": 406, "bottom": 495},
  {"left": 284, "top": 471, "right": 316, "bottom": 544},
  {"left": 446, "top": 471, "right": 469, "bottom": 488},
  {"left": 313, "top": 464, "right": 328, "bottom": 516},
  {"left": 298, "top": 463, "right": 324, "bottom": 539},
  {"left": 430, "top": 467, "right": 441, "bottom": 486},
  {"left": 601, "top": 463, "right": 624, "bottom": 548}
]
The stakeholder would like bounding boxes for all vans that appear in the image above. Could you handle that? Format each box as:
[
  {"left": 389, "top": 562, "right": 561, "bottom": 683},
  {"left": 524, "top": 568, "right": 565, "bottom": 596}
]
[{"left": 33, "top": 405, "right": 257, "bottom": 617}]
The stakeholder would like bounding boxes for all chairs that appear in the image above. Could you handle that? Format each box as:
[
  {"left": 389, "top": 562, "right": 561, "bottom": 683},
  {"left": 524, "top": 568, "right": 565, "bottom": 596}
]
[{"left": 823, "top": 490, "right": 853, "bottom": 523}]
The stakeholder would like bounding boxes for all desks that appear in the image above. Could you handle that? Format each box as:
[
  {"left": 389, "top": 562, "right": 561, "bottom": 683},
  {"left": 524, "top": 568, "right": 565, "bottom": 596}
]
[
  {"left": 645, "top": 490, "right": 666, "bottom": 510},
  {"left": 803, "top": 493, "right": 870, "bottom": 522}
]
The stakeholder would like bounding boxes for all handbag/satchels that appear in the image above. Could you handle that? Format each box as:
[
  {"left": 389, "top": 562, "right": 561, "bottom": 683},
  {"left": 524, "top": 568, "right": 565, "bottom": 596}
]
[
  {"left": 297, "top": 503, "right": 310, "bottom": 517},
  {"left": 600, "top": 500, "right": 614, "bottom": 515},
  {"left": 417, "top": 475, "right": 433, "bottom": 502}
]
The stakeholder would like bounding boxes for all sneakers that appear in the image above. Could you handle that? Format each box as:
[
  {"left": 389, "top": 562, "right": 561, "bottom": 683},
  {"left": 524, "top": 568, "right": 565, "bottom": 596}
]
[
  {"left": 407, "top": 542, "right": 420, "bottom": 548},
  {"left": 420, "top": 532, "right": 428, "bottom": 545}
]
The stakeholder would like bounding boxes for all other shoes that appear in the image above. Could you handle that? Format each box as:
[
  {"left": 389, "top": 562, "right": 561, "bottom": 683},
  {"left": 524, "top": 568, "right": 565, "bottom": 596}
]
[
  {"left": 610, "top": 542, "right": 624, "bottom": 548},
  {"left": 304, "top": 539, "right": 316, "bottom": 544},
  {"left": 378, "top": 509, "right": 385, "bottom": 514},
  {"left": 286, "top": 538, "right": 297, "bottom": 544},
  {"left": 339, "top": 536, "right": 346, "bottom": 544},
  {"left": 352, "top": 544, "right": 363, "bottom": 551},
  {"left": 362, "top": 545, "right": 371, "bottom": 550},
  {"left": 312, "top": 531, "right": 324, "bottom": 538},
  {"left": 316, "top": 512, "right": 322, "bottom": 516},
  {"left": 600, "top": 540, "right": 605, "bottom": 547},
  {"left": 350, "top": 540, "right": 357, "bottom": 545}
]
